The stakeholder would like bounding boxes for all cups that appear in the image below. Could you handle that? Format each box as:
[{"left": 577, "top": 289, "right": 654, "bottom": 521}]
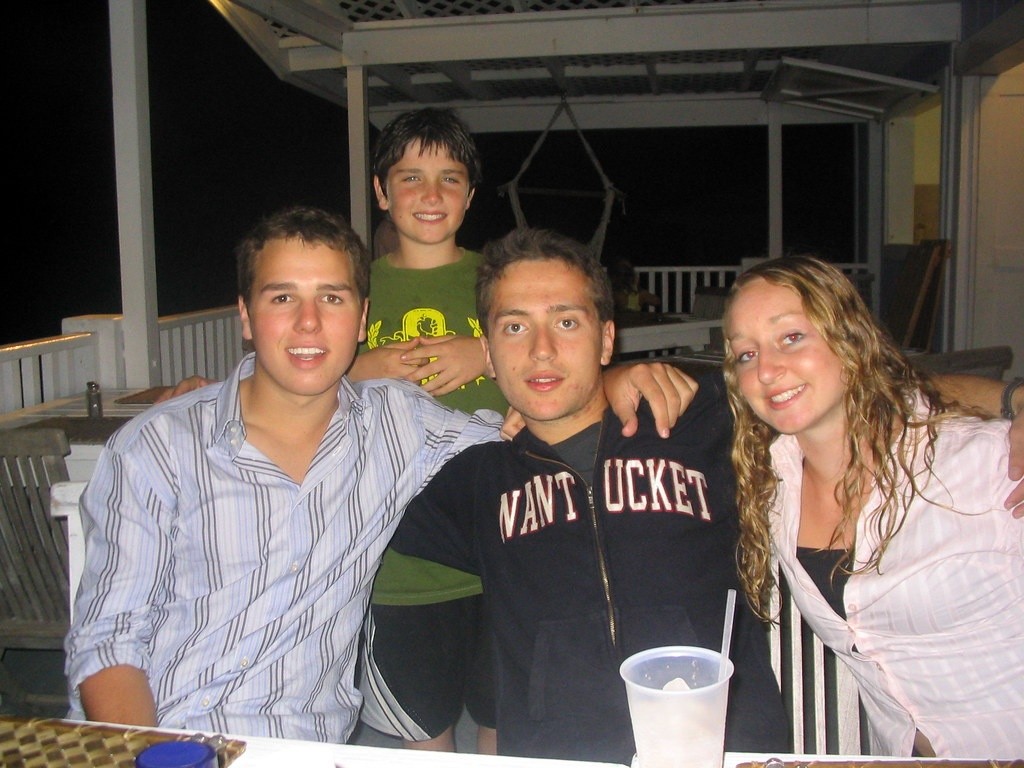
[
  {"left": 619, "top": 645, "right": 735, "bottom": 768},
  {"left": 135, "top": 740, "right": 219, "bottom": 768}
]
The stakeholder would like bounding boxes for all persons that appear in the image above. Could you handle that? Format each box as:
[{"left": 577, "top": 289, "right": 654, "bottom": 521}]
[
  {"left": 495, "top": 253, "right": 1024, "bottom": 759},
  {"left": 63, "top": 205, "right": 700, "bottom": 748},
  {"left": 154, "top": 227, "right": 1023, "bottom": 766},
  {"left": 345, "top": 109, "right": 511, "bottom": 420}
]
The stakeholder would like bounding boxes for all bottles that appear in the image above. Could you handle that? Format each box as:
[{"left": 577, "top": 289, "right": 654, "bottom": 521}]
[{"left": 85, "top": 382, "right": 103, "bottom": 418}]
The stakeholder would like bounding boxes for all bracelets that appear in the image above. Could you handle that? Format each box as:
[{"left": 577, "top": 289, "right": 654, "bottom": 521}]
[{"left": 1000, "top": 375, "right": 1024, "bottom": 419}]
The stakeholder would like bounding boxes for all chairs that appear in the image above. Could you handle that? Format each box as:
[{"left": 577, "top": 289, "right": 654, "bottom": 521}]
[
  {"left": 680, "top": 286, "right": 730, "bottom": 355},
  {"left": 0, "top": 427, "right": 71, "bottom": 650}
]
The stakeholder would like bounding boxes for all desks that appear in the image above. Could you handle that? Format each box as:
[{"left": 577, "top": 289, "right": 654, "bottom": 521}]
[
  {"left": 3, "top": 386, "right": 176, "bottom": 489},
  {"left": 611, "top": 314, "right": 723, "bottom": 353}
]
